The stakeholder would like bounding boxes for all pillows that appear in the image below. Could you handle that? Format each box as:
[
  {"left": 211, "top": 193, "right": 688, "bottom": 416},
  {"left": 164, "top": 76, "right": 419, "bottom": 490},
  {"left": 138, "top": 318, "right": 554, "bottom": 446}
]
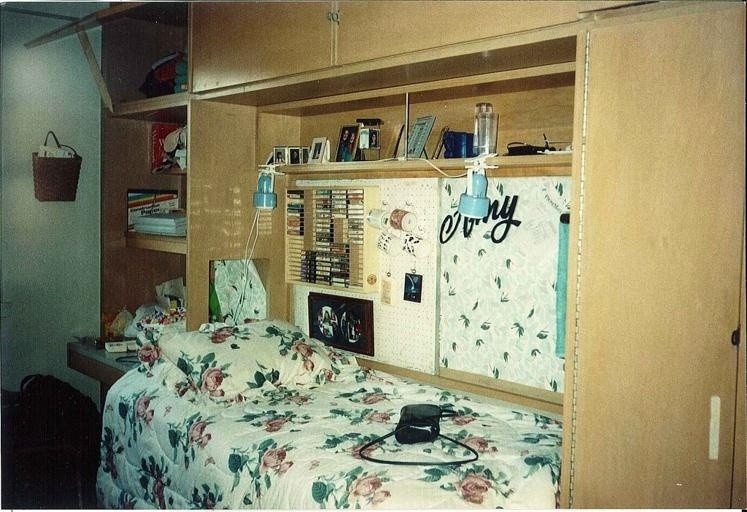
[{"left": 136, "top": 310, "right": 363, "bottom": 403}]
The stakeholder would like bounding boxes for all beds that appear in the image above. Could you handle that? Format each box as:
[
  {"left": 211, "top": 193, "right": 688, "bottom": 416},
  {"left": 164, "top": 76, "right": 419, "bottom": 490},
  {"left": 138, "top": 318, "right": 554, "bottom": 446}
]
[{"left": 94, "top": 353, "right": 565, "bottom": 507}]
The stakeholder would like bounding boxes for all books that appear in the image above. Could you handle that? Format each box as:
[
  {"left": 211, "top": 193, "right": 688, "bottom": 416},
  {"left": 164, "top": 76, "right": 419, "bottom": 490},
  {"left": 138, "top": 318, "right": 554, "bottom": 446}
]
[{"left": 134, "top": 213, "right": 187, "bottom": 234}]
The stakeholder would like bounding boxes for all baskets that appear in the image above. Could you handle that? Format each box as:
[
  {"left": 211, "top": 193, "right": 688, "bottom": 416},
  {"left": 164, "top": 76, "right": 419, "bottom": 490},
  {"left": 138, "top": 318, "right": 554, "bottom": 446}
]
[{"left": 32, "top": 152, "right": 82, "bottom": 201}]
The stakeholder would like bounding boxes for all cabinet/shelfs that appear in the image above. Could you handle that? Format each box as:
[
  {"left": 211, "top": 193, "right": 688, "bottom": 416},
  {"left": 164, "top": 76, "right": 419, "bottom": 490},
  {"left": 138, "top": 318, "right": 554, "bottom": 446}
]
[
  {"left": 96, "top": 1, "right": 257, "bottom": 363},
  {"left": 249, "top": 59, "right": 579, "bottom": 180},
  {"left": 191, "top": 3, "right": 579, "bottom": 108},
  {"left": 558, "top": 2, "right": 746, "bottom": 509}
]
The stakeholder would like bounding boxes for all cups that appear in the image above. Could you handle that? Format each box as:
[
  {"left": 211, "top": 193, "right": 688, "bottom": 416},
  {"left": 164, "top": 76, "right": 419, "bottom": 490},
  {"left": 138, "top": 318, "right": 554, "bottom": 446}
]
[{"left": 472, "top": 103, "right": 498, "bottom": 155}]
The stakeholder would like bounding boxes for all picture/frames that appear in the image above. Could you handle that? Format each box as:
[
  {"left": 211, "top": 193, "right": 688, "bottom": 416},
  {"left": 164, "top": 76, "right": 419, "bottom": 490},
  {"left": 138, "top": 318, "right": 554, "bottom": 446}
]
[
  {"left": 405, "top": 113, "right": 436, "bottom": 160},
  {"left": 333, "top": 123, "right": 360, "bottom": 162},
  {"left": 288, "top": 145, "right": 302, "bottom": 167},
  {"left": 272, "top": 144, "right": 289, "bottom": 166},
  {"left": 300, "top": 146, "right": 309, "bottom": 166},
  {"left": 367, "top": 127, "right": 381, "bottom": 148},
  {"left": 430, "top": 125, "right": 449, "bottom": 159},
  {"left": 308, "top": 136, "right": 328, "bottom": 164}
]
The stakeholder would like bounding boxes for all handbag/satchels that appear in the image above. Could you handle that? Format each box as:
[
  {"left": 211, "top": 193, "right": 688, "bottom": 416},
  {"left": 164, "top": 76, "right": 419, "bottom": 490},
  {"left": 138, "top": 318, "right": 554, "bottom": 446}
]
[{"left": 395, "top": 404, "right": 442, "bottom": 444}]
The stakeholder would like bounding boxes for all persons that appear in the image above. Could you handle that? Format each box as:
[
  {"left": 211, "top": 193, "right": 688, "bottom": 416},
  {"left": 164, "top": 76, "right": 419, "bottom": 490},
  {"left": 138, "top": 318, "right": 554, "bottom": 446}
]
[{"left": 336, "top": 129, "right": 355, "bottom": 162}]
